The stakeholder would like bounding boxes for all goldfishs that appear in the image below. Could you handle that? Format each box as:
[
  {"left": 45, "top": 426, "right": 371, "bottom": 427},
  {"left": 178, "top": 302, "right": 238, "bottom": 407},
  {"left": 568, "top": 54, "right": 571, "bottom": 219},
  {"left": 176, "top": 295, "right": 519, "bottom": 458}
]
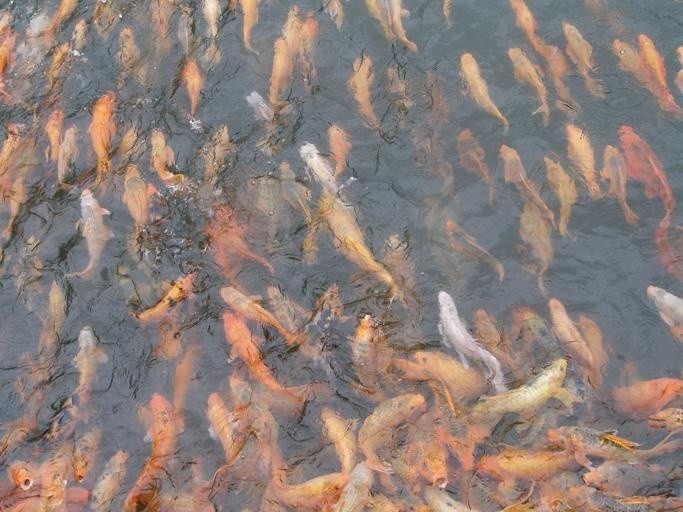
[{"left": 0, "top": 0, "right": 683, "bottom": 512}]
